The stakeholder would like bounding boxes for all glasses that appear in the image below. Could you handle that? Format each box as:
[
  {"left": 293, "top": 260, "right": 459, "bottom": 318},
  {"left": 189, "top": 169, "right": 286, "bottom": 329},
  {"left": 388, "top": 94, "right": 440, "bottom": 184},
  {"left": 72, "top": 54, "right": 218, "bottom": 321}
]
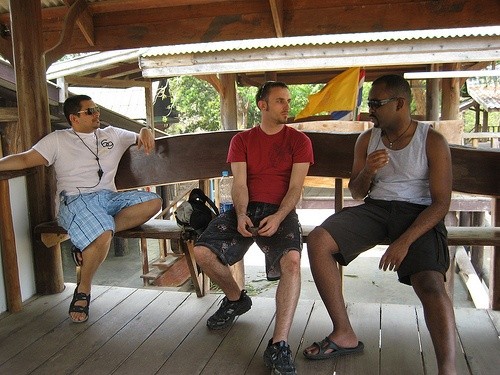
[
  {"left": 73, "top": 107, "right": 100, "bottom": 115},
  {"left": 260, "top": 81, "right": 278, "bottom": 97},
  {"left": 367, "top": 97, "right": 406, "bottom": 108}
]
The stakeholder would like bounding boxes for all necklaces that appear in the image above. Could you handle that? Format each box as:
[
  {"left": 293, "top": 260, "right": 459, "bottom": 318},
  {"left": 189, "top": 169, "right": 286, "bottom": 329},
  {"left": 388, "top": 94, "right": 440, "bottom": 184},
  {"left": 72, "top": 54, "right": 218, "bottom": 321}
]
[{"left": 385, "top": 119, "right": 413, "bottom": 148}]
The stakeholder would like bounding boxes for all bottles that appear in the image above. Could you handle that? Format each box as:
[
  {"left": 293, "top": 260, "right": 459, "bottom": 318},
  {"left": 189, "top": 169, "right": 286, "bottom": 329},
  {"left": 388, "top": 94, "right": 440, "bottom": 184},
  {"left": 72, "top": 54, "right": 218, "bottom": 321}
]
[{"left": 219, "top": 170, "right": 234, "bottom": 214}]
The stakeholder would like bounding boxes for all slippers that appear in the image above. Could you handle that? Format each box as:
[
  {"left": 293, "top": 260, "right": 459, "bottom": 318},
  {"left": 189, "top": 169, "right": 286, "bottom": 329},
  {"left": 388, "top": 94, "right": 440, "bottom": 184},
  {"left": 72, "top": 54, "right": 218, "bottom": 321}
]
[{"left": 303, "top": 337, "right": 364, "bottom": 359}]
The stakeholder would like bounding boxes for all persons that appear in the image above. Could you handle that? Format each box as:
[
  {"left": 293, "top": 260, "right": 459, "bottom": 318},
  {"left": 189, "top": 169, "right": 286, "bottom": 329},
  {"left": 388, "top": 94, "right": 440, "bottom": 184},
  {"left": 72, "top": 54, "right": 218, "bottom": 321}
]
[
  {"left": 303, "top": 74, "right": 458, "bottom": 375},
  {"left": 0, "top": 95, "right": 163, "bottom": 322},
  {"left": 193, "top": 81, "right": 314, "bottom": 375}
]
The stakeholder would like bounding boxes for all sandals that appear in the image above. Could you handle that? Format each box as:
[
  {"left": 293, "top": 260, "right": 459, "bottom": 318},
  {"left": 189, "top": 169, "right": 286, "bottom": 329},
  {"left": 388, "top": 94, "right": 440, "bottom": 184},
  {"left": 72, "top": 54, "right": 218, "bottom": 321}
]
[
  {"left": 69, "top": 285, "right": 90, "bottom": 322},
  {"left": 71, "top": 245, "right": 82, "bottom": 266}
]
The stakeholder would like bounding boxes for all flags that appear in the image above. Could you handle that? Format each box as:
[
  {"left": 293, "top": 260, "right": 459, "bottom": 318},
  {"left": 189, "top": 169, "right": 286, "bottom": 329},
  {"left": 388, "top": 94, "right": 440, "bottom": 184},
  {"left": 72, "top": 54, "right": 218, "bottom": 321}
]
[
  {"left": 295, "top": 67, "right": 360, "bottom": 120},
  {"left": 332, "top": 69, "right": 365, "bottom": 121}
]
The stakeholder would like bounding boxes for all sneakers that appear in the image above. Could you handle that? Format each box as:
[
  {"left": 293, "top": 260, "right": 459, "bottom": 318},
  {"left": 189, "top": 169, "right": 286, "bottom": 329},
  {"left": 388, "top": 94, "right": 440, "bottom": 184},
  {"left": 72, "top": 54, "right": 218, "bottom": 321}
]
[
  {"left": 207, "top": 289, "right": 252, "bottom": 329},
  {"left": 264, "top": 338, "right": 297, "bottom": 375}
]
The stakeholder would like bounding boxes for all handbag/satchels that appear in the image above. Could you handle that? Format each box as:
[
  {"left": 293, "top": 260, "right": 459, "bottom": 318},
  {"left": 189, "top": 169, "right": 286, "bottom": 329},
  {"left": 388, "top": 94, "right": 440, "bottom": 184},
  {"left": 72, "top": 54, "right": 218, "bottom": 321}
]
[{"left": 174, "top": 186, "right": 220, "bottom": 236}]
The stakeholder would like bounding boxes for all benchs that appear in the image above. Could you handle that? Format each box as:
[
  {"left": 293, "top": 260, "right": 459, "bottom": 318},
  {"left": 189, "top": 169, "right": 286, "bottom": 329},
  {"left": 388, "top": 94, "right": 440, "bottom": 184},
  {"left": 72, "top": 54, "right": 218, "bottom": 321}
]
[{"left": 30, "top": 129, "right": 500, "bottom": 311}]
